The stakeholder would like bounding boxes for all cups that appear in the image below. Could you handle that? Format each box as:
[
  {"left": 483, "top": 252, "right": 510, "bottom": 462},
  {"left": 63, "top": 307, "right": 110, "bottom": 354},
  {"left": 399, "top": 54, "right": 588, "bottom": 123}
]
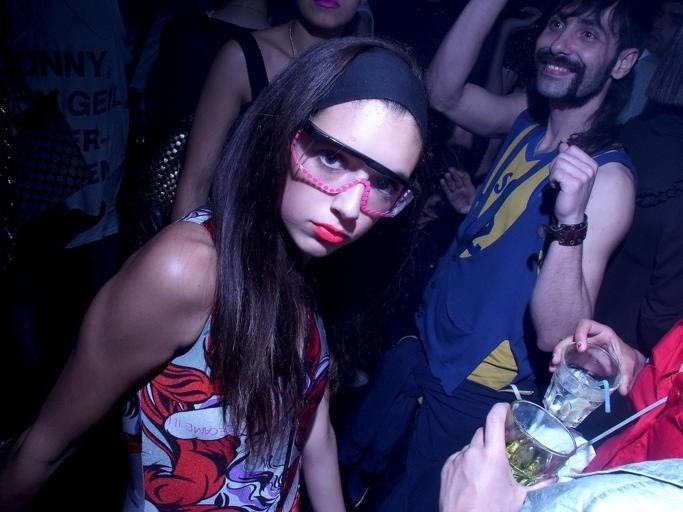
[{"left": 504, "top": 342, "right": 622, "bottom": 487}]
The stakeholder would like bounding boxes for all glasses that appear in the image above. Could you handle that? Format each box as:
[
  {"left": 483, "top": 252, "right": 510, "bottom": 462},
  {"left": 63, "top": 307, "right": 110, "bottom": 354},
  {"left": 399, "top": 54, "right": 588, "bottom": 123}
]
[{"left": 290, "top": 116, "right": 422, "bottom": 221}]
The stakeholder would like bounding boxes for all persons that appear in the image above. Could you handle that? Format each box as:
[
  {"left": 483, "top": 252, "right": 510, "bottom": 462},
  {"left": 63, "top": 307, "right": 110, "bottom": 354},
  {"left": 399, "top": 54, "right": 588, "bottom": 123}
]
[{"left": 0, "top": 0, "right": 682, "bottom": 511}]
